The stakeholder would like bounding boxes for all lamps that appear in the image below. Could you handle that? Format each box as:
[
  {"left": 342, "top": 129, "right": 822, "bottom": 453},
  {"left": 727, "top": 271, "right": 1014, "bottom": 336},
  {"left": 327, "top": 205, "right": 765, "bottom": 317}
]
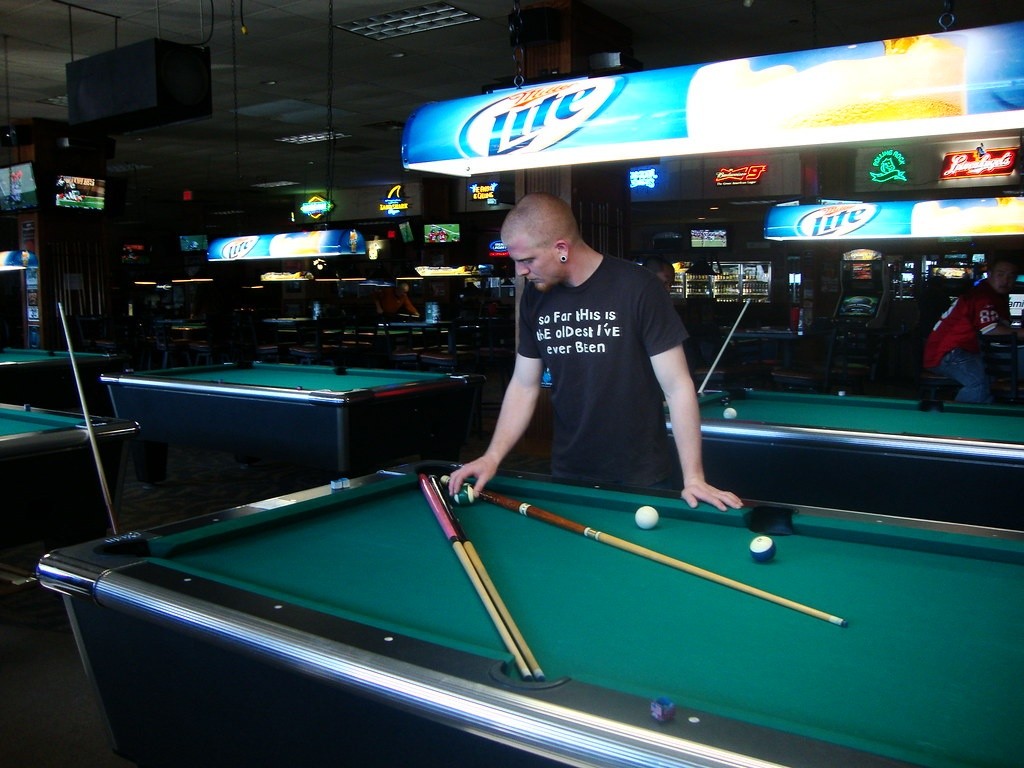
[
  {"left": 205, "top": 0, "right": 368, "bottom": 262},
  {"left": 400, "top": 1, "right": 1024, "bottom": 242}
]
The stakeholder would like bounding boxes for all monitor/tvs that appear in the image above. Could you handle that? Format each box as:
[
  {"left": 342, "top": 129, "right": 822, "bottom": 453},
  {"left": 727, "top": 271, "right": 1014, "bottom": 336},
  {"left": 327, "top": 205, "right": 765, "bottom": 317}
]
[
  {"left": 56, "top": 176, "right": 105, "bottom": 210},
  {"left": 691, "top": 229, "right": 727, "bottom": 248},
  {"left": 424, "top": 223, "right": 461, "bottom": 243},
  {"left": 0, "top": 160, "right": 42, "bottom": 216},
  {"left": 179, "top": 235, "right": 209, "bottom": 251}
]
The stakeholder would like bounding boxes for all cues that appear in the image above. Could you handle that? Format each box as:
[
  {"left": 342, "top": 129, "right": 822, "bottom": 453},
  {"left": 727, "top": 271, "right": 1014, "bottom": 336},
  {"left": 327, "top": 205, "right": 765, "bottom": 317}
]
[
  {"left": 698, "top": 297, "right": 751, "bottom": 394},
  {"left": 57, "top": 301, "right": 122, "bottom": 536},
  {"left": 439, "top": 473, "right": 850, "bottom": 628},
  {"left": 416, "top": 470, "right": 548, "bottom": 682}
]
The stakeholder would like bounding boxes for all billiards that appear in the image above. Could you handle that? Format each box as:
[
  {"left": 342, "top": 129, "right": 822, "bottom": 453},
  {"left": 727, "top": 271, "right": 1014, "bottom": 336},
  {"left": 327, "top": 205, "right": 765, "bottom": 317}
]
[
  {"left": 721, "top": 396, "right": 731, "bottom": 407},
  {"left": 749, "top": 535, "right": 776, "bottom": 562},
  {"left": 635, "top": 506, "right": 659, "bottom": 529},
  {"left": 453, "top": 483, "right": 475, "bottom": 504},
  {"left": 296, "top": 386, "right": 302, "bottom": 390},
  {"left": 648, "top": 696, "right": 677, "bottom": 724},
  {"left": 723, "top": 407, "right": 737, "bottom": 419}
]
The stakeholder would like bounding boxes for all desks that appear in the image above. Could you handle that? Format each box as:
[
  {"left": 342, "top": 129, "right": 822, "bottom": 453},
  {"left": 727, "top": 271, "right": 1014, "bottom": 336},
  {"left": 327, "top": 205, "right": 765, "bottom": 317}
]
[{"left": 0, "top": 318, "right": 1024, "bottom": 768}]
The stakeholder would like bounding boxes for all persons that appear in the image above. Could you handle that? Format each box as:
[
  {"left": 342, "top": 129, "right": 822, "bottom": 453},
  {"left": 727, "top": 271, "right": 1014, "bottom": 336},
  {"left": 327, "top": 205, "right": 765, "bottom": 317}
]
[
  {"left": 450, "top": 192, "right": 744, "bottom": 511},
  {"left": 375, "top": 283, "right": 419, "bottom": 316},
  {"left": 924, "top": 256, "right": 1024, "bottom": 405},
  {"left": 640, "top": 257, "right": 676, "bottom": 296}
]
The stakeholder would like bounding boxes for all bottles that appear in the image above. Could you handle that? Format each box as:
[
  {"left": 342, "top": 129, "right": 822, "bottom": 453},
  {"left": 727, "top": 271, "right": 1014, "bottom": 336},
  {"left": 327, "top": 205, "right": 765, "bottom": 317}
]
[{"left": 670, "top": 267, "right": 769, "bottom": 304}]
